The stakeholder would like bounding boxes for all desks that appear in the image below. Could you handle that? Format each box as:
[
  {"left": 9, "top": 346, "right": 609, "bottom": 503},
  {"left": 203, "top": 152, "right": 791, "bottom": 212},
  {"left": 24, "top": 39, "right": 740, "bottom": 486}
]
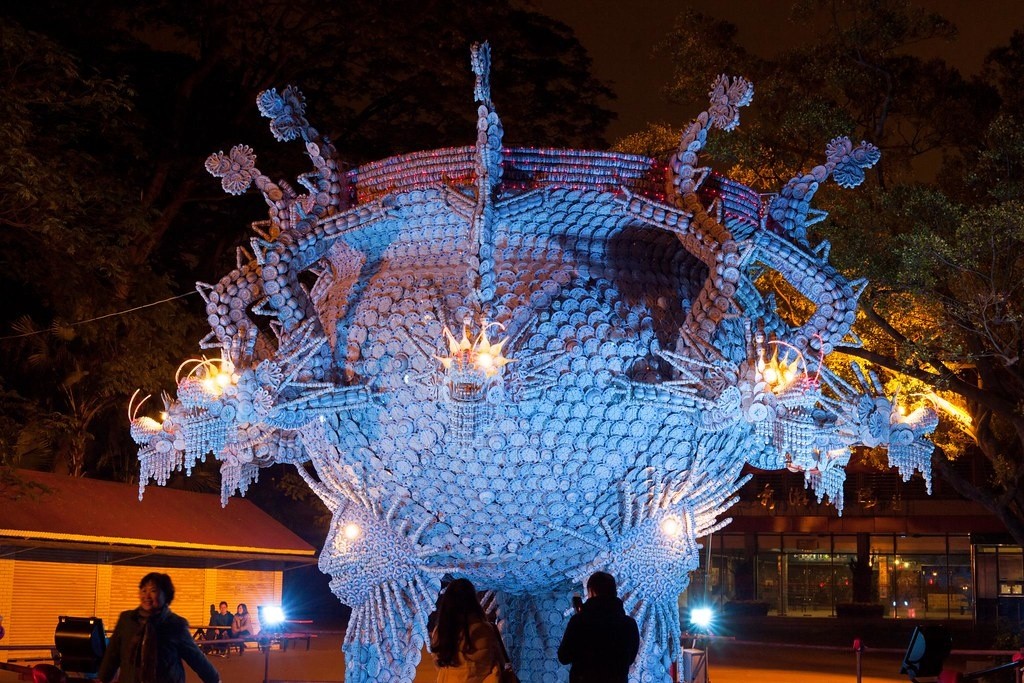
[
  {"left": 284, "top": 618, "right": 313, "bottom": 652},
  {"left": 680, "top": 632, "right": 736, "bottom": 683},
  {"left": 187, "top": 625, "right": 231, "bottom": 655}
]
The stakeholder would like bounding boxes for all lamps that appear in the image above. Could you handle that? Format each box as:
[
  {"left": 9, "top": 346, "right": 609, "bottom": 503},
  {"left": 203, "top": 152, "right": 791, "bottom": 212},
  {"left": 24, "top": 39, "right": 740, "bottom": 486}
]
[
  {"left": 54, "top": 615, "right": 108, "bottom": 674},
  {"left": 899, "top": 624, "right": 953, "bottom": 683}
]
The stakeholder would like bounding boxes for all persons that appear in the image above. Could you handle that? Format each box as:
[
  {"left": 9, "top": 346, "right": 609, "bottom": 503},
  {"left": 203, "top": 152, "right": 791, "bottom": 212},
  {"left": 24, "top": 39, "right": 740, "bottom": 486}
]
[
  {"left": 231, "top": 603, "right": 254, "bottom": 656},
  {"left": 557, "top": 571, "right": 640, "bottom": 683},
  {"left": 212, "top": 601, "right": 234, "bottom": 657},
  {"left": 426, "top": 578, "right": 512, "bottom": 683},
  {"left": 93, "top": 571, "right": 221, "bottom": 683}
]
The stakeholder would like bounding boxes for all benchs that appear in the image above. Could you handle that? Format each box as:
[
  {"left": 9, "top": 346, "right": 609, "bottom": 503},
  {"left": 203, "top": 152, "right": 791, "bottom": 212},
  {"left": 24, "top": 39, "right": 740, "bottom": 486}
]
[
  {"left": 194, "top": 638, "right": 245, "bottom": 656},
  {"left": 928, "top": 593, "right": 969, "bottom": 615},
  {"left": 238, "top": 632, "right": 318, "bottom": 652}
]
